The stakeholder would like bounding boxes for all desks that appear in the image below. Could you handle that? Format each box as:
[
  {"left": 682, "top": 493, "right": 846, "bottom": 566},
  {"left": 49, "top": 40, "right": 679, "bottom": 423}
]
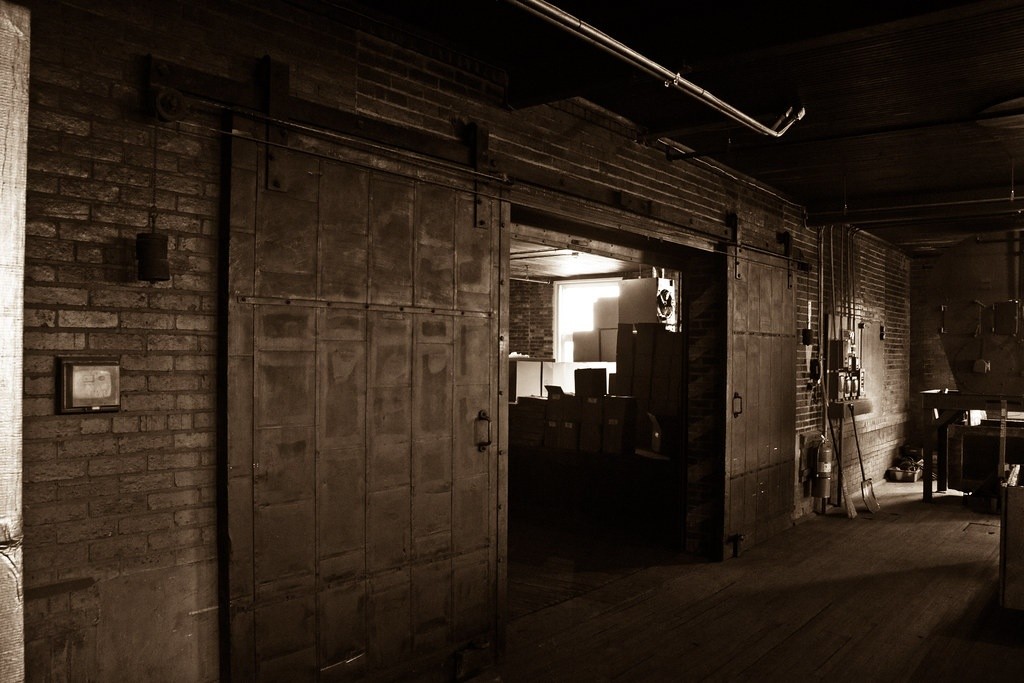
[{"left": 920, "top": 389, "right": 1024, "bottom": 504}]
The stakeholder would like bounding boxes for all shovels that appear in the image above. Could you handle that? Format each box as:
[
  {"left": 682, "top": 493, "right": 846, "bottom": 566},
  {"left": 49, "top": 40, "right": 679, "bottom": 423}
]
[{"left": 849, "top": 402, "right": 881, "bottom": 515}]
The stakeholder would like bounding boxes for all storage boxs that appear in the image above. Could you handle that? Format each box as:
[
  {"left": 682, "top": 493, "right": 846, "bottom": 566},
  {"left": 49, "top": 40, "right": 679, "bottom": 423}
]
[{"left": 543, "top": 323, "right": 681, "bottom": 456}]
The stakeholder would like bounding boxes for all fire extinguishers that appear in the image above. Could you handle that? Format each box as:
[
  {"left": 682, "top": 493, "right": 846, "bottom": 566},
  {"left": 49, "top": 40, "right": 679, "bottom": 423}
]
[{"left": 813, "top": 433, "right": 833, "bottom": 499}]
